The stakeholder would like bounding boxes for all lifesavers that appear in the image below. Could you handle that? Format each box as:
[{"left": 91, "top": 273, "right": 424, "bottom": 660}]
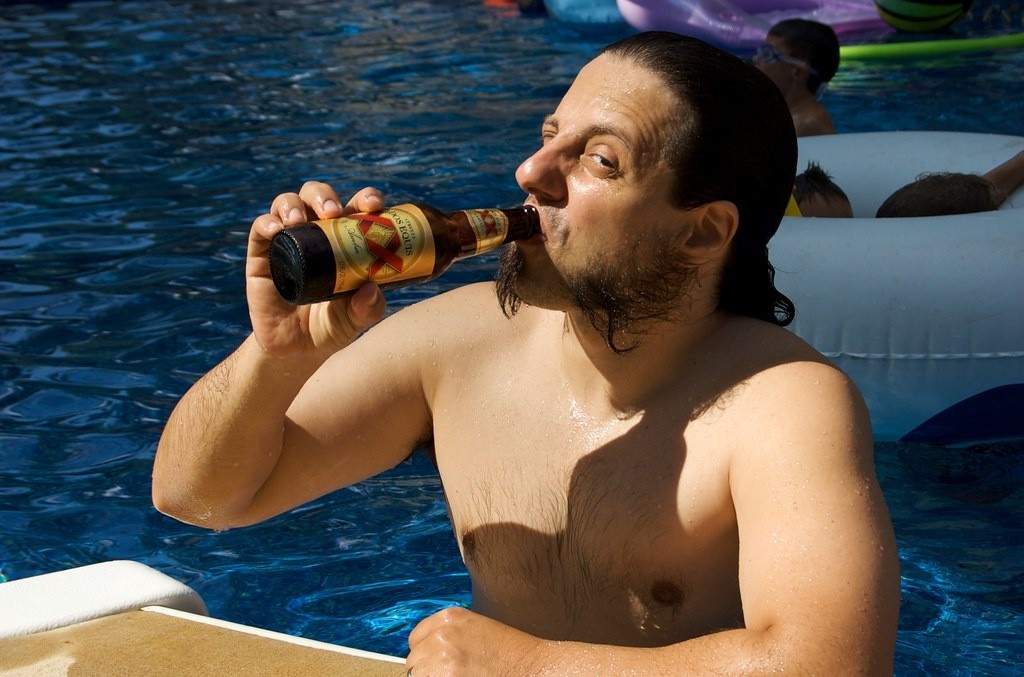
[{"left": 771, "top": 127, "right": 1024, "bottom": 452}]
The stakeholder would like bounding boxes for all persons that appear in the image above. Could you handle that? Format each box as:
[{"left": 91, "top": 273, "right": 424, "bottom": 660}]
[
  {"left": 753, "top": 19, "right": 1024, "bottom": 218},
  {"left": 154, "top": 30, "right": 898, "bottom": 677}
]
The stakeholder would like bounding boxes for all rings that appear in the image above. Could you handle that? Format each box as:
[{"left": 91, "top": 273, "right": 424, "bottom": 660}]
[{"left": 407, "top": 666, "right": 414, "bottom": 677}]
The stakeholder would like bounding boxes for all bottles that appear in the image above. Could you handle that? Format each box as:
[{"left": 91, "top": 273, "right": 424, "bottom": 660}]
[{"left": 270, "top": 205, "right": 540, "bottom": 304}]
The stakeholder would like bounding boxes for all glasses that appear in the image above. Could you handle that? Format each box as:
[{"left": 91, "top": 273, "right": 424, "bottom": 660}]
[{"left": 758, "top": 42, "right": 816, "bottom": 74}]
